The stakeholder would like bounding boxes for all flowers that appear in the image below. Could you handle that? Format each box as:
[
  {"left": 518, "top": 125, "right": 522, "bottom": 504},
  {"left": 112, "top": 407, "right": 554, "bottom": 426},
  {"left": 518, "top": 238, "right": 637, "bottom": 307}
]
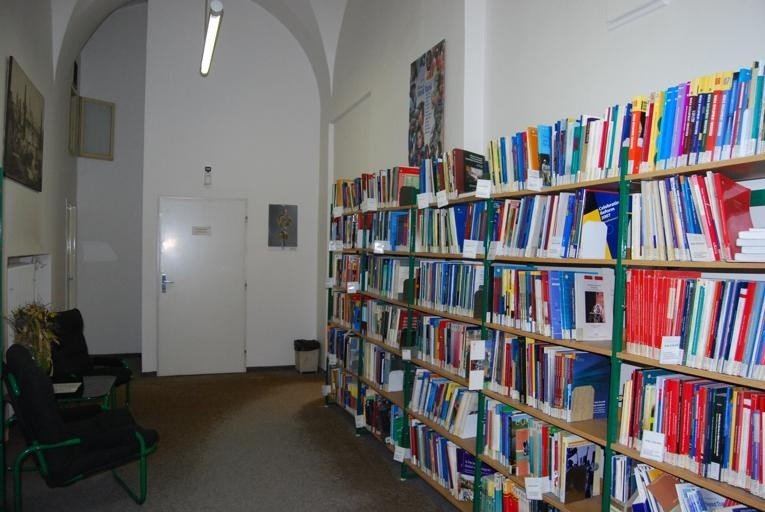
[{"left": 4, "top": 297, "right": 60, "bottom": 368}]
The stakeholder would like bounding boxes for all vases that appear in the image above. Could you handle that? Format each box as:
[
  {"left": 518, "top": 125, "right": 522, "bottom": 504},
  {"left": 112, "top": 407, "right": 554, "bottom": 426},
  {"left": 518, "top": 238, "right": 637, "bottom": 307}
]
[{"left": 32, "top": 350, "right": 52, "bottom": 375}]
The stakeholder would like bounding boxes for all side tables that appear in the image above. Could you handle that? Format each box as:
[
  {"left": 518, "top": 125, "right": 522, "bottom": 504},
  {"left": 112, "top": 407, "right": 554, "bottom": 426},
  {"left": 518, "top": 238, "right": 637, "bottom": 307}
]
[{"left": 4, "top": 375, "right": 119, "bottom": 448}]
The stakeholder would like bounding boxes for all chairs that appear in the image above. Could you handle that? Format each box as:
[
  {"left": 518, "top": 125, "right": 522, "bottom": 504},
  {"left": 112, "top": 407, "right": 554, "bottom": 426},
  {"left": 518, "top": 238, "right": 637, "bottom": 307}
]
[
  {"left": 48, "top": 308, "right": 133, "bottom": 413},
  {"left": 4, "top": 344, "right": 159, "bottom": 512}
]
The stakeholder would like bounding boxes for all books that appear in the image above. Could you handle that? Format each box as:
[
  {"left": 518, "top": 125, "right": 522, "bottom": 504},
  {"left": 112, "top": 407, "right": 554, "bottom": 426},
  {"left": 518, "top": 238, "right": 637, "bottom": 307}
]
[
  {"left": 480, "top": 63, "right": 765, "bottom": 512},
  {"left": 324, "top": 149, "right": 485, "bottom": 501}
]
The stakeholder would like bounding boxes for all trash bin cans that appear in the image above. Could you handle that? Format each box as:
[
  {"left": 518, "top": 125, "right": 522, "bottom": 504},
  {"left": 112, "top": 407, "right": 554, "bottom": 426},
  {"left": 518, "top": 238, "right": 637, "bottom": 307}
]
[{"left": 294, "top": 339, "right": 320, "bottom": 373}]
